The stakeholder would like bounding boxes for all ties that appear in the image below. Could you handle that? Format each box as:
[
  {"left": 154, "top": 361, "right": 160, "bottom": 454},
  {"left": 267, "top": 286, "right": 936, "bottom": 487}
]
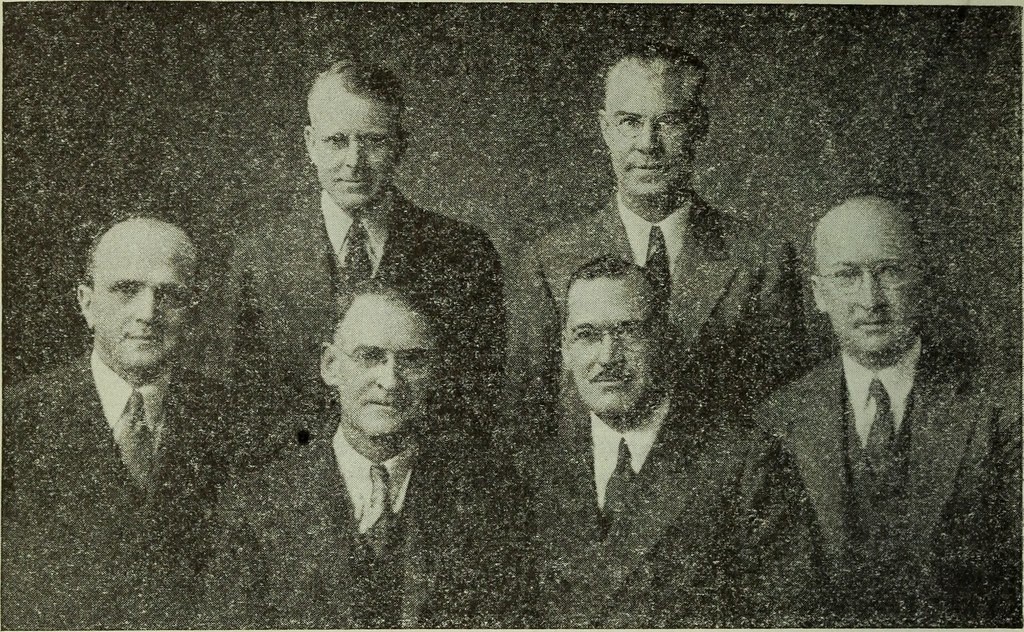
[
  {"left": 600, "top": 437, "right": 638, "bottom": 543},
  {"left": 359, "top": 466, "right": 398, "bottom": 568},
  {"left": 342, "top": 222, "right": 374, "bottom": 296},
  {"left": 862, "top": 380, "right": 895, "bottom": 506},
  {"left": 119, "top": 393, "right": 155, "bottom": 495},
  {"left": 645, "top": 225, "right": 672, "bottom": 328}
]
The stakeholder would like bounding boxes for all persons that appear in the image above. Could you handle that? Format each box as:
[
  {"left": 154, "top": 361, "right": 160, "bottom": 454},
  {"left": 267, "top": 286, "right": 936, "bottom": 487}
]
[
  {"left": 220, "top": 57, "right": 506, "bottom": 486},
  {"left": 203, "top": 281, "right": 539, "bottom": 629},
  {"left": 524, "top": 253, "right": 830, "bottom": 627},
  {"left": 513, "top": 40, "right": 803, "bottom": 499},
  {"left": 748, "top": 187, "right": 1021, "bottom": 629},
  {"left": 1, "top": 212, "right": 271, "bottom": 629}
]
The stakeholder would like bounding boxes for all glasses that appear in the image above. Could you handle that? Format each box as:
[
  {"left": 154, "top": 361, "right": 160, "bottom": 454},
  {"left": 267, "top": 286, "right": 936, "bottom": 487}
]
[
  {"left": 340, "top": 345, "right": 439, "bottom": 370},
  {"left": 609, "top": 113, "right": 689, "bottom": 138},
  {"left": 812, "top": 261, "right": 924, "bottom": 293},
  {"left": 564, "top": 321, "right": 663, "bottom": 354}
]
